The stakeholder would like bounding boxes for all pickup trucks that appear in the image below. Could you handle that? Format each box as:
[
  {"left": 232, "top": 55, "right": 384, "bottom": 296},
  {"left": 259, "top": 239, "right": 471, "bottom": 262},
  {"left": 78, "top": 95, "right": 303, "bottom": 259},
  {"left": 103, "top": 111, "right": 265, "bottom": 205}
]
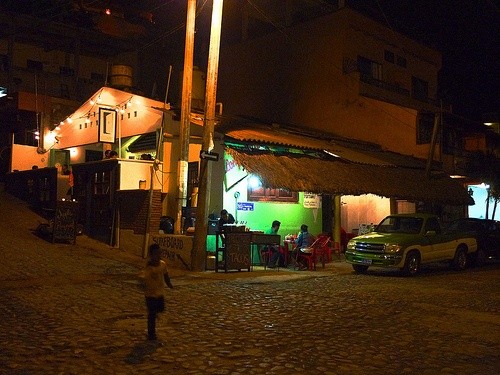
[{"left": 345, "top": 213, "right": 478, "bottom": 276}]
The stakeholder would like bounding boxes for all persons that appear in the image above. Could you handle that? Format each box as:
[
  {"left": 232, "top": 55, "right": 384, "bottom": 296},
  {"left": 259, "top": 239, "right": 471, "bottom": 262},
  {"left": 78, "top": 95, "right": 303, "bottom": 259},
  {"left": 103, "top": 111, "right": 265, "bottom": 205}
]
[
  {"left": 218, "top": 210, "right": 235, "bottom": 261},
  {"left": 62, "top": 163, "right": 70, "bottom": 175},
  {"left": 261, "top": 220, "right": 287, "bottom": 268},
  {"left": 136, "top": 244, "right": 173, "bottom": 348},
  {"left": 101, "top": 149, "right": 118, "bottom": 161},
  {"left": 290, "top": 224, "right": 314, "bottom": 271}
]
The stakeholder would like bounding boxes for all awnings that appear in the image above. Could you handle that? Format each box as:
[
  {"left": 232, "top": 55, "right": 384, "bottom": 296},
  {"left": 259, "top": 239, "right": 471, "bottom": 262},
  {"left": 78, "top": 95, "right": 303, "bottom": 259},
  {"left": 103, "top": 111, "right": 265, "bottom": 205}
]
[{"left": 222, "top": 147, "right": 478, "bottom": 205}]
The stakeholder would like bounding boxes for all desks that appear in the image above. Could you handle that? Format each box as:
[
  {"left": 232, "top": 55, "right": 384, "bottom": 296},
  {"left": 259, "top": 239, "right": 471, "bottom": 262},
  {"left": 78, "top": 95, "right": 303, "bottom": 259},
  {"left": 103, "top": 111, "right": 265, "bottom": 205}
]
[
  {"left": 215, "top": 230, "right": 281, "bottom": 272},
  {"left": 283, "top": 240, "right": 296, "bottom": 267}
]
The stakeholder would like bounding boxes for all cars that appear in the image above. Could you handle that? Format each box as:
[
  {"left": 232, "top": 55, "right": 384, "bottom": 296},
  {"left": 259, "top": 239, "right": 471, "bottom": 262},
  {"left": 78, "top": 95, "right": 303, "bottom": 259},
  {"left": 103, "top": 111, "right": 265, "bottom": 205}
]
[{"left": 448, "top": 218, "right": 500, "bottom": 267}]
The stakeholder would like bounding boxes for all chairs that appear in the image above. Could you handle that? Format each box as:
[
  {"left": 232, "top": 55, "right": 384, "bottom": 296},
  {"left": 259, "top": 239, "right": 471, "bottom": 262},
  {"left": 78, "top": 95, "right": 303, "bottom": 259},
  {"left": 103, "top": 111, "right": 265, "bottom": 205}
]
[{"left": 260, "top": 227, "right": 355, "bottom": 270}]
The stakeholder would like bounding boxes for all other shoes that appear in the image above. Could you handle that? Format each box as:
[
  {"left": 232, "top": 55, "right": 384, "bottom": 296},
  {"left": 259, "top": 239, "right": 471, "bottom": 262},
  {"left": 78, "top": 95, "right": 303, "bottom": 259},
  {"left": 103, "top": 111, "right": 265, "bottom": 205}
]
[
  {"left": 298, "top": 264, "right": 305, "bottom": 270},
  {"left": 267, "top": 263, "right": 276, "bottom": 268}
]
